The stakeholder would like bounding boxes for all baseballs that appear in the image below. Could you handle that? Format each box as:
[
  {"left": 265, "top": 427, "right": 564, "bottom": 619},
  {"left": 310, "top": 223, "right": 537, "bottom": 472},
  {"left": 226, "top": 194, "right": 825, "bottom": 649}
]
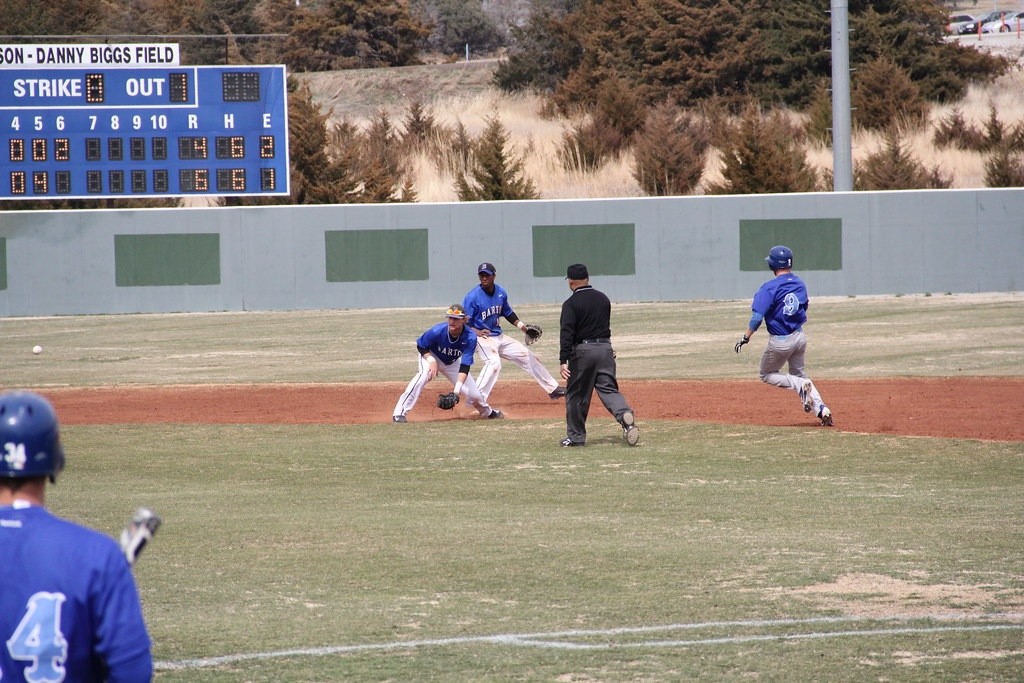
[{"left": 32, "top": 345, "right": 43, "bottom": 355}]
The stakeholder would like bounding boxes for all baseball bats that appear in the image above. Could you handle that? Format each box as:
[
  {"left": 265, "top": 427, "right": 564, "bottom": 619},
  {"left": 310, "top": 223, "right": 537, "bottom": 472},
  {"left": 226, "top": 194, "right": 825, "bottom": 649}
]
[{"left": 118, "top": 508, "right": 162, "bottom": 566}]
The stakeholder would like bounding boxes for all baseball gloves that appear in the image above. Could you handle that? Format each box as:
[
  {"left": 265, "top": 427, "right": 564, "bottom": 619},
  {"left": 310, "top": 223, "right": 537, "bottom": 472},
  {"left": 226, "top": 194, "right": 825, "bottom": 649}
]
[
  {"left": 525, "top": 324, "right": 543, "bottom": 345},
  {"left": 436, "top": 392, "right": 459, "bottom": 410}
]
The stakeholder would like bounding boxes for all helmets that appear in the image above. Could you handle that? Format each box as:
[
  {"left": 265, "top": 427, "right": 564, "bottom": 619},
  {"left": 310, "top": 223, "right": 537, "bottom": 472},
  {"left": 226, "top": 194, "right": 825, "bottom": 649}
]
[
  {"left": 763, "top": 245, "right": 793, "bottom": 270},
  {"left": 0, "top": 390, "right": 66, "bottom": 483}
]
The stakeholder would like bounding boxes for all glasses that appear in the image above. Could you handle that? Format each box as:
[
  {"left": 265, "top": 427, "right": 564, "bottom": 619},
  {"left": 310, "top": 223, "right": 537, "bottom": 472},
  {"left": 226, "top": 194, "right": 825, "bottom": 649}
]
[{"left": 447, "top": 308, "right": 465, "bottom": 316}]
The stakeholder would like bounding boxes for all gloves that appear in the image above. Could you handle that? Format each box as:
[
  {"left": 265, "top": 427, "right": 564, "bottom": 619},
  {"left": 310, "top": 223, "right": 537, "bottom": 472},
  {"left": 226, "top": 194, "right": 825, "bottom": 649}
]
[{"left": 734, "top": 335, "right": 750, "bottom": 353}]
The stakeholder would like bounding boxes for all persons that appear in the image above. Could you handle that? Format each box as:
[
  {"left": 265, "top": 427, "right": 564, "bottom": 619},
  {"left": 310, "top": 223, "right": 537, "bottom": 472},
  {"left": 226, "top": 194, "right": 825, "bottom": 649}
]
[
  {"left": 392, "top": 305, "right": 503, "bottom": 424},
  {"left": 558, "top": 264, "right": 639, "bottom": 447},
  {"left": 463, "top": 263, "right": 568, "bottom": 418},
  {"left": 735, "top": 246, "right": 834, "bottom": 426},
  {"left": 1, "top": 396, "right": 158, "bottom": 681}
]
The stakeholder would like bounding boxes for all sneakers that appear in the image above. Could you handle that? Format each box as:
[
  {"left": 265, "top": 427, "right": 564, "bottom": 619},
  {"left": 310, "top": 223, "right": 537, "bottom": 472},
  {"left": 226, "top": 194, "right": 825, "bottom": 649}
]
[
  {"left": 560, "top": 437, "right": 585, "bottom": 446},
  {"left": 620, "top": 411, "right": 640, "bottom": 445},
  {"left": 800, "top": 380, "right": 813, "bottom": 413},
  {"left": 821, "top": 406, "right": 833, "bottom": 426},
  {"left": 549, "top": 386, "right": 567, "bottom": 399},
  {"left": 393, "top": 416, "right": 408, "bottom": 422},
  {"left": 488, "top": 409, "right": 504, "bottom": 419}
]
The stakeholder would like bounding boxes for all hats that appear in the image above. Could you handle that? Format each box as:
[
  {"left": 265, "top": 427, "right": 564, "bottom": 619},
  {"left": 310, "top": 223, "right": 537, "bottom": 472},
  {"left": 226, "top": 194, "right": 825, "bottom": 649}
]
[
  {"left": 564, "top": 264, "right": 589, "bottom": 281},
  {"left": 478, "top": 262, "right": 496, "bottom": 275},
  {"left": 445, "top": 304, "right": 466, "bottom": 320}
]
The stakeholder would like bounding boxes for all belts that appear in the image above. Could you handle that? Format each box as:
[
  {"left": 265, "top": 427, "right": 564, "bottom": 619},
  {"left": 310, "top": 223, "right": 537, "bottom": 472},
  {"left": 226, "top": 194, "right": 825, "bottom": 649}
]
[{"left": 579, "top": 338, "right": 612, "bottom": 343}]
